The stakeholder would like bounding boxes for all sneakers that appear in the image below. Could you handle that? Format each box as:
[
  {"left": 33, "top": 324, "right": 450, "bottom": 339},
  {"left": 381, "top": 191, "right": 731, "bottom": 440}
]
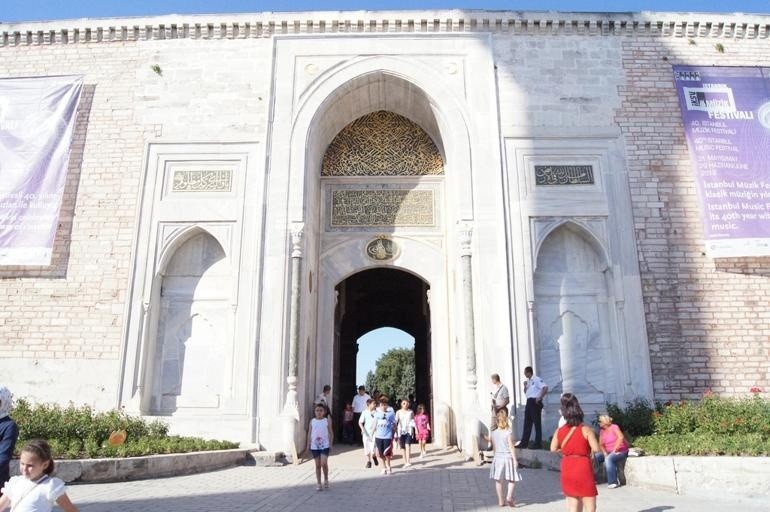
[
  {"left": 367, "top": 462, "right": 371, "bottom": 468},
  {"left": 373, "top": 456, "right": 378, "bottom": 465}
]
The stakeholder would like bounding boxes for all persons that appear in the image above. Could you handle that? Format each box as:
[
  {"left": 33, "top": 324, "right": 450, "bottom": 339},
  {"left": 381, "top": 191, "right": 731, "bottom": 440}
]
[
  {"left": 343, "top": 385, "right": 432, "bottom": 477},
  {"left": 488, "top": 410, "right": 523, "bottom": 507},
  {"left": 0, "top": 438, "right": 76, "bottom": 512},
  {"left": 587, "top": 414, "right": 629, "bottom": 489},
  {"left": 513, "top": 366, "right": 549, "bottom": 449},
  {"left": 487, "top": 373, "right": 509, "bottom": 449},
  {"left": 305, "top": 403, "right": 334, "bottom": 491},
  {"left": 550, "top": 393, "right": 601, "bottom": 512},
  {"left": 311, "top": 384, "right": 332, "bottom": 419},
  {"left": 0, "top": 388, "right": 18, "bottom": 491}
]
